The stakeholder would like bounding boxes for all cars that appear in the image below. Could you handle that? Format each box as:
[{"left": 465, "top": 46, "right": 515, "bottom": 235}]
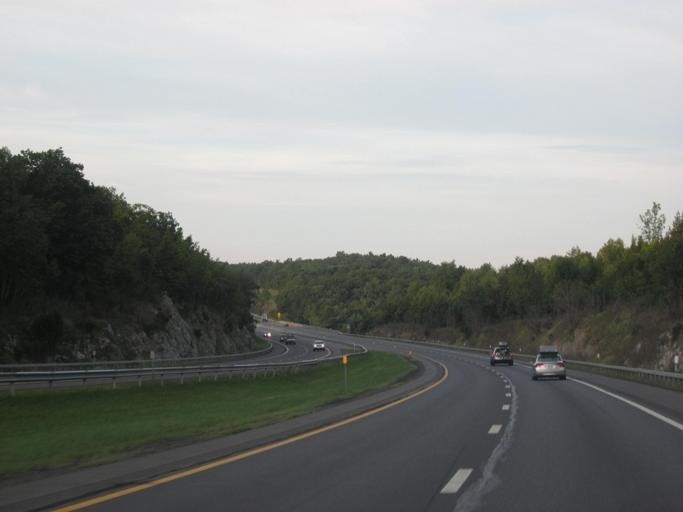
[
  {"left": 279, "top": 332, "right": 296, "bottom": 347},
  {"left": 531, "top": 350, "right": 567, "bottom": 381},
  {"left": 489, "top": 347, "right": 513, "bottom": 367},
  {"left": 263, "top": 330, "right": 271, "bottom": 338}
]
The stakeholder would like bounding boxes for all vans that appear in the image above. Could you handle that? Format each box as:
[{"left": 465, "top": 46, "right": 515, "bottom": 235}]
[{"left": 312, "top": 340, "right": 325, "bottom": 351}]
